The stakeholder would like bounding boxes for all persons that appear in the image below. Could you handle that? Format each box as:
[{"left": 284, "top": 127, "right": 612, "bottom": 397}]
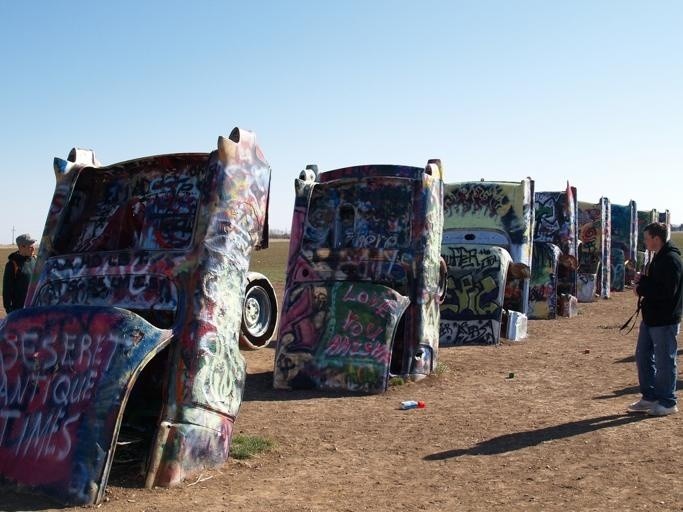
[
  {"left": 2, "top": 234, "right": 38, "bottom": 315},
  {"left": 626, "top": 222, "right": 683, "bottom": 416}
]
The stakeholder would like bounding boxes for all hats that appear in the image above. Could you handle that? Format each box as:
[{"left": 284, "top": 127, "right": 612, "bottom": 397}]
[{"left": 15, "top": 234, "right": 35, "bottom": 246}]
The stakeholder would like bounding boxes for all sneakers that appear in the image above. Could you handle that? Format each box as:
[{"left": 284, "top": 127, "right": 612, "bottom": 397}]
[
  {"left": 648, "top": 404, "right": 678, "bottom": 416},
  {"left": 629, "top": 400, "right": 658, "bottom": 410}
]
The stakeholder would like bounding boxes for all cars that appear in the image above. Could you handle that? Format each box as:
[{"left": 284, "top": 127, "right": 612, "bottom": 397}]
[
  {"left": 658, "top": 209, "right": 670, "bottom": 227},
  {"left": 1, "top": 127, "right": 280, "bottom": 503},
  {"left": 576, "top": 195, "right": 612, "bottom": 303},
  {"left": 610, "top": 198, "right": 639, "bottom": 293},
  {"left": 527, "top": 178, "right": 582, "bottom": 319},
  {"left": 435, "top": 174, "right": 535, "bottom": 347},
  {"left": 270, "top": 155, "right": 447, "bottom": 395},
  {"left": 637, "top": 207, "right": 659, "bottom": 273}
]
